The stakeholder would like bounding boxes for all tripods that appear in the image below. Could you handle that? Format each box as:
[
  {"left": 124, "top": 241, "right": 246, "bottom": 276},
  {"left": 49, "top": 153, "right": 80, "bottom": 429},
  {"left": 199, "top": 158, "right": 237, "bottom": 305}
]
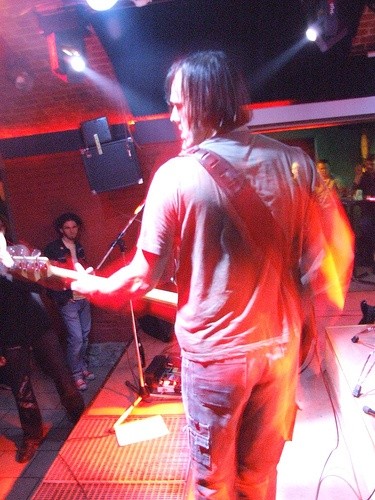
[{"left": 96, "top": 202, "right": 183, "bottom": 434}]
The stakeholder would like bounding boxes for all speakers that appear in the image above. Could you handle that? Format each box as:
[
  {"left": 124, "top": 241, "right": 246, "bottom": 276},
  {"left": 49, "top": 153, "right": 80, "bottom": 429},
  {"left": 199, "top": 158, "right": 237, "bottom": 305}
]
[
  {"left": 80, "top": 117, "right": 113, "bottom": 147},
  {"left": 80, "top": 137, "right": 143, "bottom": 195}
]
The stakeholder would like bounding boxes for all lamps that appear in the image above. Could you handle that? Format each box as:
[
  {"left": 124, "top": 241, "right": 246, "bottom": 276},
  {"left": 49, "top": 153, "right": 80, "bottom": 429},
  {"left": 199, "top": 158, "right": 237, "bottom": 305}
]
[
  {"left": 47, "top": 30, "right": 87, "bottom": 85},
  {"left": 304, "top": 6, "right": 347, "bottom": 53}
]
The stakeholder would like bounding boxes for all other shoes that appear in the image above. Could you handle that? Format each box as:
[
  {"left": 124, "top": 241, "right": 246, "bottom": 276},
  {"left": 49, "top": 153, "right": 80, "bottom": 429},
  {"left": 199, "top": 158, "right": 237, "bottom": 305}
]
[
  {"left": 82, "top": 370, "right": 95, "bottom": 379},
  {"left": 15, "top": 435, "right": 43, "bottom": 463},
  {"left": 74, "top": 378, "right": 88, "bottom": 390}
]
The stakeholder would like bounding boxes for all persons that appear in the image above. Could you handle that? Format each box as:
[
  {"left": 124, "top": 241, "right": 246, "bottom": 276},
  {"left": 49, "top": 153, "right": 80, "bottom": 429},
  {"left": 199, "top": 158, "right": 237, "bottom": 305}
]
[
  {"left": 46, "top": 213, "right": 96, "bottom": 391},
  {"left": 0, "top": 52, "right": 374, "bottom": 500}
]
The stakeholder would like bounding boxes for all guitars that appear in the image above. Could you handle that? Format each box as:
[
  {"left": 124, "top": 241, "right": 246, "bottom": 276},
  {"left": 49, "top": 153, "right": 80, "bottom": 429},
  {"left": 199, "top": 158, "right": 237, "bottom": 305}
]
[{"left": 1, "top": 244, "right": 316, "bottom": 375}]
